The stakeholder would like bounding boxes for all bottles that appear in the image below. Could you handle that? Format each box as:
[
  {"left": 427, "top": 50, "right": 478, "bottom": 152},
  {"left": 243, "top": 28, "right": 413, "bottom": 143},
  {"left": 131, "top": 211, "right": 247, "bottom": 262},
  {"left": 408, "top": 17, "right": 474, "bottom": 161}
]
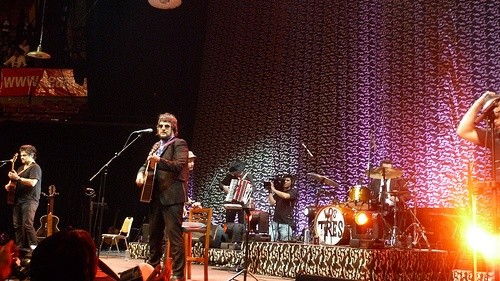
[
  {"left": 304, "top": 230, "right": 310, "bottom": 244},
  {"left": 391, "top": 226, "right": 398, "bottom": 246},
  {"left": 408, "top": 233, "right": 412, "bottom": 248}
]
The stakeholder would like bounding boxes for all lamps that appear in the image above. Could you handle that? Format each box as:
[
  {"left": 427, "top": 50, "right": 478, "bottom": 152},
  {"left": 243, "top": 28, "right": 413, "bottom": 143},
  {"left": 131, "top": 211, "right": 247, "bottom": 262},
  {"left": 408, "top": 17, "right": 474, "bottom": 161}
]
[
  {"left": 25, "top": 0, "right": 51, "bottom": 60},
  {"left": 248, "top": 211, "right": 269, "bottom": 235},
  {"left": 352, "top": 210, "right": 384, "bottom": 240},
  {"left": 223, "top": 222, "right": 245, "bottom": 243}
]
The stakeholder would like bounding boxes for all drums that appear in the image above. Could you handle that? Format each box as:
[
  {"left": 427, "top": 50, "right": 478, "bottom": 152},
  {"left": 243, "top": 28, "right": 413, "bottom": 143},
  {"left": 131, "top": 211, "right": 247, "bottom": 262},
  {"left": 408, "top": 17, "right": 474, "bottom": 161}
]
[
  {"left": 314, "top": 203, "right": 355, "bottom": 246},
  {"left": 346, "top": 185, "right": 375, "bottom": 211}
]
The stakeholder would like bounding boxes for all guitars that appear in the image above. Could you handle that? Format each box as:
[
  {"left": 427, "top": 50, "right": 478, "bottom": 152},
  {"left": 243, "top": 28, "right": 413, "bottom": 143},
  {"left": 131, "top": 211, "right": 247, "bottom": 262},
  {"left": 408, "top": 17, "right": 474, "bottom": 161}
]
[
  {"left": 35, "top": 185, "right": 60, "bottom": 243},
  {"left": 140, "top": 141, "right": 162, "bottom": 203},
  {"left": 5, "top": 152, "right": 19, "bottom": 192}
]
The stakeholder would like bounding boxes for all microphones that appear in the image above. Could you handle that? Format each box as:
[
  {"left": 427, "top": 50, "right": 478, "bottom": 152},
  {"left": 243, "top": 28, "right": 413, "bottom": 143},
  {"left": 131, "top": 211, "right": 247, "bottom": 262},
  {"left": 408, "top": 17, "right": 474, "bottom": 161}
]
[
  {"left": 475, "top": 98, "right": 500, "bottom": 125},
  {"left": 302, "top": 144, "right": 314, "bottom": 157},
  {"left": 2, "top": 159, "right": 14, "bottom": 162},
  {"left": 134, "top": 128, "right": 154, "bottom": 134}
]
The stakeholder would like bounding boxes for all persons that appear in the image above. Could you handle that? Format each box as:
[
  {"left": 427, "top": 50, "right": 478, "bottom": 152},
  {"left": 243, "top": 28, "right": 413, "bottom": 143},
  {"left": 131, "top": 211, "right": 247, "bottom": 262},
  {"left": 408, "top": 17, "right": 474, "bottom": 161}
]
[
  {"left": 4, "top": 145, "right": 42, "bottom": 254},
  {"left": 267, "top": 174, "right": 297, "bottom": 242},
  {"left": 456, "top": 90, "right": 500, "bottom": 201},
  {"left": 0, "top": 226, "right": 99, "bottom": 281},
  {"left": 220, "top": 160, "right": 255, "bottom": 250},
  {"left": 367, "top": 159, "right": 413, "bottom": 248},
  {"left": 135, "top": 112, "right": 202, "bottom": 281}
]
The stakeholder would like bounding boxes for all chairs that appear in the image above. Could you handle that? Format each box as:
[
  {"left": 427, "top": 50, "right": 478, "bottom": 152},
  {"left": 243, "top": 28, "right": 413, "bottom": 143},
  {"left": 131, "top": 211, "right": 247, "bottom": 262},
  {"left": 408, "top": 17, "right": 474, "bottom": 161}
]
[{"left": 96, "top": 216, "right": 134, "bottom": 260}]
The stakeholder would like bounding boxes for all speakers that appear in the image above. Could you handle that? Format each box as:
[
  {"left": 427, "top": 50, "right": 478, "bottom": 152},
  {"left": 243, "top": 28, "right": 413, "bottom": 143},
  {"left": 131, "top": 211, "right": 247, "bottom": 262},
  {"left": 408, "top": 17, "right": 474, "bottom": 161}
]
[
  {"left": 119, "top": 262, "right": 159, "bottom": 281},
  {"left": 142, "top": 223, "right": 150, "bottom": 242},
  {"left": 194, "top": 223, "right": 223, "bottom": 247}
]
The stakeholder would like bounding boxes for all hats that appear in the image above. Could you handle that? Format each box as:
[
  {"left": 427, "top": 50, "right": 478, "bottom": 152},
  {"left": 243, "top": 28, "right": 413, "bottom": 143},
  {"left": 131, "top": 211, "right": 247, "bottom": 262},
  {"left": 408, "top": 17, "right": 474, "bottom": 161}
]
[
  {"left": 188, "top": 151, "right": 197, "bottom": 158},
  {"left": 229, "top": 161, "right": 245, "bottom": 173}
]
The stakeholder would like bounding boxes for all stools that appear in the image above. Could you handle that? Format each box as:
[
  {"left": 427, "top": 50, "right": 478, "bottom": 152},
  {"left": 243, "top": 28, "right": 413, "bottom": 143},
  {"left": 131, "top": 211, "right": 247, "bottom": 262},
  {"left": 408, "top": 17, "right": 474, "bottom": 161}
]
[{"left": 166, "top": 207, "right": 213, "bottom": 281}]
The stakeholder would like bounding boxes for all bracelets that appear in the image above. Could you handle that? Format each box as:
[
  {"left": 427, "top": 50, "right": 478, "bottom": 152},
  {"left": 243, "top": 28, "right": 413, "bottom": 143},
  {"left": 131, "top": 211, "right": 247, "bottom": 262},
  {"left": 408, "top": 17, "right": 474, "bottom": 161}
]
[{"left": 18, "top": 176, "right": 21, "bottom": 184}]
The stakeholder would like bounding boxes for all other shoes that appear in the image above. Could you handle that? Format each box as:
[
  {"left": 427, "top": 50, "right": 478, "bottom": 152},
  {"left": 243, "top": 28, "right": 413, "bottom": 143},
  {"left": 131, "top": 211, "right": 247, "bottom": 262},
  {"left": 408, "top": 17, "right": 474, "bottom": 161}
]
[{"left": 171, "top": 275, "right": 186, "bottom": 281}]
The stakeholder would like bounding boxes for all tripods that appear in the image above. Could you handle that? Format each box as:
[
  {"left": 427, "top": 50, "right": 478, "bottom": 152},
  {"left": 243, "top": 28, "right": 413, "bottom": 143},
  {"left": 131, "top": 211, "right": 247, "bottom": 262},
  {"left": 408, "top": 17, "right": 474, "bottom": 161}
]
[
  {"left": 228, "top": 209, "right": 259, "bottom": 281},
  {"left": 381, "top": 195, "right": 408, "bottom": 249}
]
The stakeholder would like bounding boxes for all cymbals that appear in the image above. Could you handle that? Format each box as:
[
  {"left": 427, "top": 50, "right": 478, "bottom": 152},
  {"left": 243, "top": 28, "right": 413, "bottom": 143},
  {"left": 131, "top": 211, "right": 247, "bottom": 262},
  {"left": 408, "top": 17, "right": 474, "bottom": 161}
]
[
  {"left": 380, "top": 190, "right": 411, "bottom": 195},
  {"left": 365, "top": 166, "right": 404, "bottom": 180},
  {"left": 306, "top": 173, "right": 339, "bottom": 187}
]
